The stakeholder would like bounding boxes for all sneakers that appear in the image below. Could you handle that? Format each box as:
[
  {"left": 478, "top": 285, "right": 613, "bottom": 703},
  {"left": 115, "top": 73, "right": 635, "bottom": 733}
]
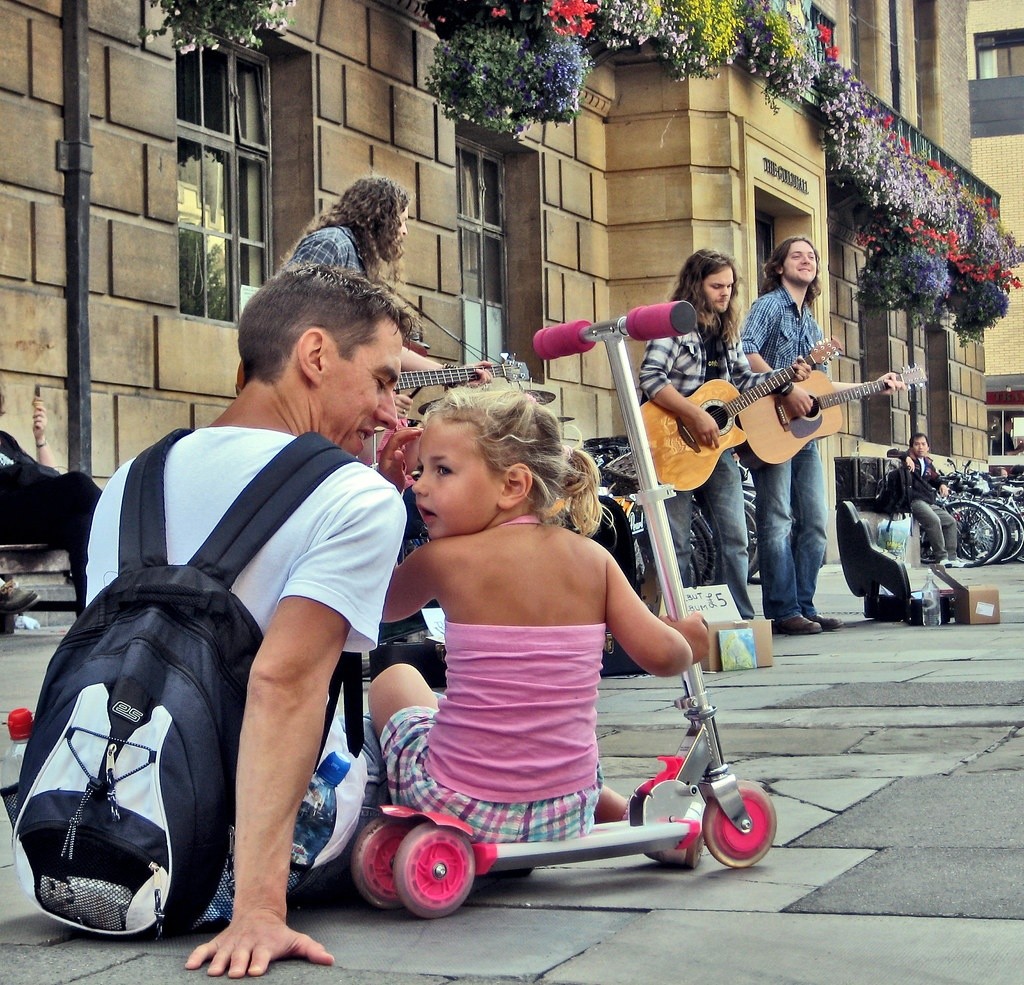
[
  {"left": 949, "top": 560, "right": 967, "bottom": 568},
  {"left": 940, "top": 559, "right": 952, "bottom": 568}
]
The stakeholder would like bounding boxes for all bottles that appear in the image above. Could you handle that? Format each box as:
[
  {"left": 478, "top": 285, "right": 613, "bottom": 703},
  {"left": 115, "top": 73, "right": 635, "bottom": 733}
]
[
  {"left": 201, "top": 750, "right": 352, "bottom": 923},
  {"left": 0, "top": 707, "right": 34, "bottom": 827},
  {"left": 922, "top": 570, "right": 942, "bottom": 627}
]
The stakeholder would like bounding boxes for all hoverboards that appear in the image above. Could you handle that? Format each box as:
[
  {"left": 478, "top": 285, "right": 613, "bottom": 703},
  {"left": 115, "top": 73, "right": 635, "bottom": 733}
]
[{"left": 349, "top": 296, "right": 779, "bottom": 919}]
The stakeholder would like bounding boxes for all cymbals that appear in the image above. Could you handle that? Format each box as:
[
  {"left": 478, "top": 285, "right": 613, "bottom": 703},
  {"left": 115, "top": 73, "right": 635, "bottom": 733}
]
[{"left": 418, "top": 388, "right": 557, "bottom": 415}]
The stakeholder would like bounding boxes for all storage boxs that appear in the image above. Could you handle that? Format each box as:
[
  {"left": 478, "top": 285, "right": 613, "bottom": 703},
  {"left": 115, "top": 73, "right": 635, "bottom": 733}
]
[
  {"left": 699, "top": 617, "right": 774, "bottom": 672},
  {"left": 929, "top": 564, "right": 1001, "bottom": 625}
]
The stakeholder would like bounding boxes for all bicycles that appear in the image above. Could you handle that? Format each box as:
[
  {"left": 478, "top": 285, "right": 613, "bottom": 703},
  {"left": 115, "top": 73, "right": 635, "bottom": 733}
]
[
  {"left": 583, "top": 433, "right": 767, "bottom": 586},
  {"left": 935, "top": 459, "right": 1024, "bottom": 569}
]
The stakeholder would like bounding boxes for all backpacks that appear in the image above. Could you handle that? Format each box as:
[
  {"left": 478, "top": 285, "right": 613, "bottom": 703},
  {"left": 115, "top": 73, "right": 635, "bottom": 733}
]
[
  {"left": 1, "top": 428, "right": 365, "bottom": 943},
  {"left": 877, "top": 465, "right": 913, "bottom": 536}
]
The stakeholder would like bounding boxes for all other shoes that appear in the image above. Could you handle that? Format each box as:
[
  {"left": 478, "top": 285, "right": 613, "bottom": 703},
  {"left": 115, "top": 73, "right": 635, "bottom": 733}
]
[
  {"left": 477, "top": 867, "right": 534, "bottom": 884},
  {"left": 641, "top": 837, "right": 703, "bottom": 867},
  {"left": 0, "top": 580, "right": 41, "bottom": 615}
]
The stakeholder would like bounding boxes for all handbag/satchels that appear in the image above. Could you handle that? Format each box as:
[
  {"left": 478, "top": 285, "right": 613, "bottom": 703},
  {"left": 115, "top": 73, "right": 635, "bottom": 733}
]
[{"left": 876, "top": 511, "right": 911, "bottom": 563}]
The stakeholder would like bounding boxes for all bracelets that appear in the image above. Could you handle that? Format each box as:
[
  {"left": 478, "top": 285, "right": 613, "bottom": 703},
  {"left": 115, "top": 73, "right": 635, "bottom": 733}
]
[
  {"left": 36, "top": 439, "right": 48, "bottom": 448},
  {"left": 781, "top": 383, "right": 794, "bottom": 396}
]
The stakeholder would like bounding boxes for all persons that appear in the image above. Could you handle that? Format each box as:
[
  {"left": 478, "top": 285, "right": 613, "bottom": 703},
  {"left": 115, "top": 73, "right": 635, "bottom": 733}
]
[
  {"left": 740, "top": 237, "right": 906, "bottom": 635},
  {"left": 281, "top": 175, "right": 494, "bottom": 418},
  {"left": 991, "top": 415, "right": 1024, "bottom": 456},
  {"left": 86, "top": 264, "right": 414, "bottom": 979},
  {"left": 0, "top": 578, "right": 41, "bottom": 615},
  {"left": 368, "top": 385, "right": 709, "bottom": 870},
  {"left": 639, "top": 248, "right": 812, "bottom": 619},
  {"left": 887, "top": 432, "right": 970, "bottom": 568},
  {"left": 0, "top": 392, "right": 103, "bottom": 620}
]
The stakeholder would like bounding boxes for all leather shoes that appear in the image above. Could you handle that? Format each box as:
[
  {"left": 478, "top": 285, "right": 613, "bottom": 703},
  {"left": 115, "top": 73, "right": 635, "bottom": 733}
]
[
  {"left": 772, "top": 615, "right": 823, "bottom": 635},
  {"left": 807, "top": 615, "right": 844, "bottom": 629}
]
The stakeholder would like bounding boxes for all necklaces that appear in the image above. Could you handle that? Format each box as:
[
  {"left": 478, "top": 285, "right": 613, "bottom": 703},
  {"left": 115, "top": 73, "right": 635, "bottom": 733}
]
[{"left": 706, "top": 338, "right": 718, "bottom": 366}]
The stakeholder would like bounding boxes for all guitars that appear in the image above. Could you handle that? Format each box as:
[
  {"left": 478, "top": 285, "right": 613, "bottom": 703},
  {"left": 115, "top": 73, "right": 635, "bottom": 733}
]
[
  {"left": 639, "top": 334, "right": 846, "bottom": 494},
  {"left": 393, "top": 348, "right": 533, "bottom": 393},
  {"left": 738, "top": 360, "right": 929, "bottom": 467}
]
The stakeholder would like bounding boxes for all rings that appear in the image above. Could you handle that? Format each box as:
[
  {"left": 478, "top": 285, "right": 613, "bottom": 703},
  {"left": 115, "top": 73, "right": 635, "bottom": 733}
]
[{"left": 403, "top": 409, "right": 406, "bottom": 414}]
